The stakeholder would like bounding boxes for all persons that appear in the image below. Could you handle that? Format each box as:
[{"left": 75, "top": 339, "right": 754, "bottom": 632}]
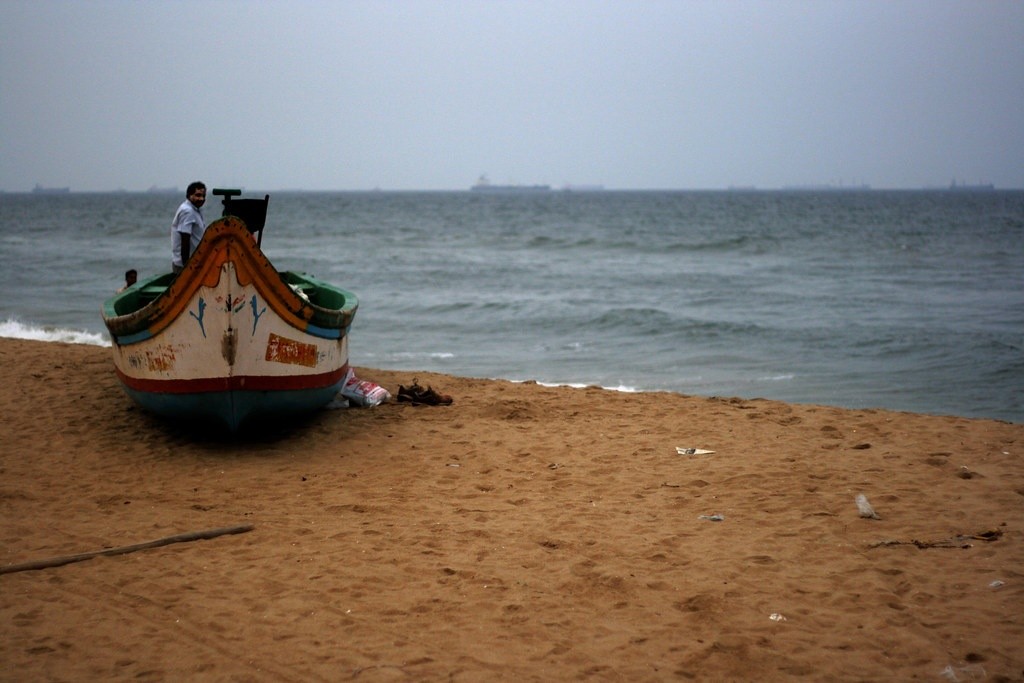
[{"left": 170, "top": 181, "right": 207, "bottom": 275}]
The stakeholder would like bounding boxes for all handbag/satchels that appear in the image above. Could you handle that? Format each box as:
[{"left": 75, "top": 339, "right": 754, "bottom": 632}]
[{"left": 340, "top": 368, "right": 392, "bottom": 410}]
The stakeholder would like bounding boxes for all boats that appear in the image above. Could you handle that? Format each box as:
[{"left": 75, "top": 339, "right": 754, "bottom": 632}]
[{"left": 101, "top": 185, "right": 359, "bottom": 449}]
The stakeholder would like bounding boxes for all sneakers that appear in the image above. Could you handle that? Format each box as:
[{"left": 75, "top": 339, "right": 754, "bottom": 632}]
[{"left": 397, "top": 377, "right": 453, "bottom": 406}]
